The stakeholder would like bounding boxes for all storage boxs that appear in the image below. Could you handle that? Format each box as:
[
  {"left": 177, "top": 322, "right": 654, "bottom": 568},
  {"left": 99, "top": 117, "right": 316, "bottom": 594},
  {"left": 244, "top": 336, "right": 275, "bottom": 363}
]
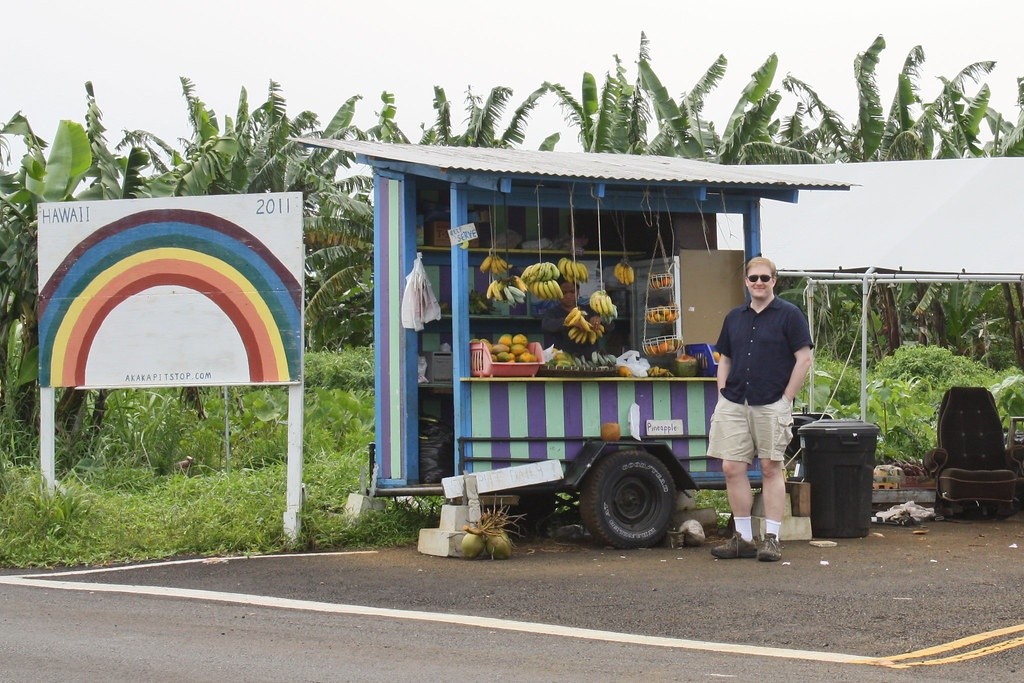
[
  {"left": 425, "top": 214, "right": 480, "bottom": 249},
  {"left": 686, "top": 343, "right": 722, "bottom": 378},
  {"left": 470, "top": 341, "right": 545, "bottom": 378}
]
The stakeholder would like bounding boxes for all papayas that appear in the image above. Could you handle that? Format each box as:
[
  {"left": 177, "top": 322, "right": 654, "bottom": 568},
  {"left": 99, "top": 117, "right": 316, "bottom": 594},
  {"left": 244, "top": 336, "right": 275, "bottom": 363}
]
[{"left": 619, "top": 366, "right": 631, "bottom": 376}]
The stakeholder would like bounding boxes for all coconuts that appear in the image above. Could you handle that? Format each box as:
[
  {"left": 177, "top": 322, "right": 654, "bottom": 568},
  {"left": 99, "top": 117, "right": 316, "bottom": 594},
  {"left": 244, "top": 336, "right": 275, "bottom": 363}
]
[
  {"left": 460, "top": 528, "right": 513, "bottom": 559},
  {"left": 600, "top": 423, "right": 620, "bottom": 442}
]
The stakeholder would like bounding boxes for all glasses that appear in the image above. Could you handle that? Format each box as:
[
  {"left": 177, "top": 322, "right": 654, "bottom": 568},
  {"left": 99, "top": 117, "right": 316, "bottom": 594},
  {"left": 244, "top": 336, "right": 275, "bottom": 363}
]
[{"left": 746, "top": 275, "right": 774, "bottom": 282}]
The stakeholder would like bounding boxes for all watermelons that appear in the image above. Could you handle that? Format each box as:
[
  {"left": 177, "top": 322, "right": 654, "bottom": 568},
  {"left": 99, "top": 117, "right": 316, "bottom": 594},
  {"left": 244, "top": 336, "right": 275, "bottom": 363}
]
[{"left": 673, "top": 354, "right": 698, "bottom": 377}]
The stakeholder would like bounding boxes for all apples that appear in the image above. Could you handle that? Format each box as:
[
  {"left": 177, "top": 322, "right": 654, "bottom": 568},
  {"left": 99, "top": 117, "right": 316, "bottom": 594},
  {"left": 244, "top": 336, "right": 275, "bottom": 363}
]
[
  {"left": 647, "top": 308, "right": 677, "bottom": 323},
  {"left": 644, "top": 338, "right": 681, "bottom": 354},
  {"left": 652, "top": 277, "right": 672, "bottom": 287}
]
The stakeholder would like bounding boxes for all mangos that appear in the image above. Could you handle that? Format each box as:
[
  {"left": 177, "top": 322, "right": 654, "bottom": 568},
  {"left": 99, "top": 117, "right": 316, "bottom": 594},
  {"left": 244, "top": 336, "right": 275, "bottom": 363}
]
[
  {"left": 550, "top": 347, "right": 577, "bottom": 366},
  {"left": 713, "top": 352, "right": 720, "bottom": 362},
  {"left": 470, "top": 333, "right": 539, "bottom": 362}
]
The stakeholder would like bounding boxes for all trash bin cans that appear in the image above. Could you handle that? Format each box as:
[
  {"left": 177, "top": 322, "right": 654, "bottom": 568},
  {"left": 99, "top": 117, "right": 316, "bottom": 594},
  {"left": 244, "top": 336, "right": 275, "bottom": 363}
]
[{"left": 800, "top": 418, "right": 880, "bottom": 538}]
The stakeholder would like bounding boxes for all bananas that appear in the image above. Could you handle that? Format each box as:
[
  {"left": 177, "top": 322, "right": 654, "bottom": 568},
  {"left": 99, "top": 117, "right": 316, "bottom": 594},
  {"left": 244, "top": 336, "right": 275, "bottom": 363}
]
[
  {"left": 469, "top": 292, "right": 489, "bottom": 313},
  {"left": 479, "top": 254, "right": 634, "bottom": 343},
  {"left": 648, "top": 365, "right": 673, "bottom": 377}
]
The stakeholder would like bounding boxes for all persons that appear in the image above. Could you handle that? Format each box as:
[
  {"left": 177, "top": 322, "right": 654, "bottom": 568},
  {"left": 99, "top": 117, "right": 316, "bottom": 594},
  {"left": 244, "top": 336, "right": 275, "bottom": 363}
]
[
  {"left": 542, "top": 273, "right": 599, "bottom": 360},
  {"left": 707, "top": 256, "right": 813, "bottom": 560}
]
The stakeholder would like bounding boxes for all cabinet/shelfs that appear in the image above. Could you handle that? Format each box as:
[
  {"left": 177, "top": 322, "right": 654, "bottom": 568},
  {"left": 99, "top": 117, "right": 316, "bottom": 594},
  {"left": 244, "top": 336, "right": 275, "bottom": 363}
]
[{"left": 416, "top": 245, "right": 645, "bottom": 320}]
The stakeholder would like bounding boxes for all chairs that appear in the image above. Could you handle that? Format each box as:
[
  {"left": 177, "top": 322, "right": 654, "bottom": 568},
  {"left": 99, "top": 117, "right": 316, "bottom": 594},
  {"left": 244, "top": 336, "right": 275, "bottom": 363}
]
[{"left": 923, "top": 386, "right": 1024, "bottom": 517}]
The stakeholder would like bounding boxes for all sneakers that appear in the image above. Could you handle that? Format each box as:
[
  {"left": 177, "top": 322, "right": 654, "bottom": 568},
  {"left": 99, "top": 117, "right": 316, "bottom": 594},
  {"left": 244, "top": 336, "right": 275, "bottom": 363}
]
[
  {"left": 710, "top": 531, "right": 758, "bottom": 559},
  {"left": 758, "top": 533, "right": 782, "bottom": 561}
]
